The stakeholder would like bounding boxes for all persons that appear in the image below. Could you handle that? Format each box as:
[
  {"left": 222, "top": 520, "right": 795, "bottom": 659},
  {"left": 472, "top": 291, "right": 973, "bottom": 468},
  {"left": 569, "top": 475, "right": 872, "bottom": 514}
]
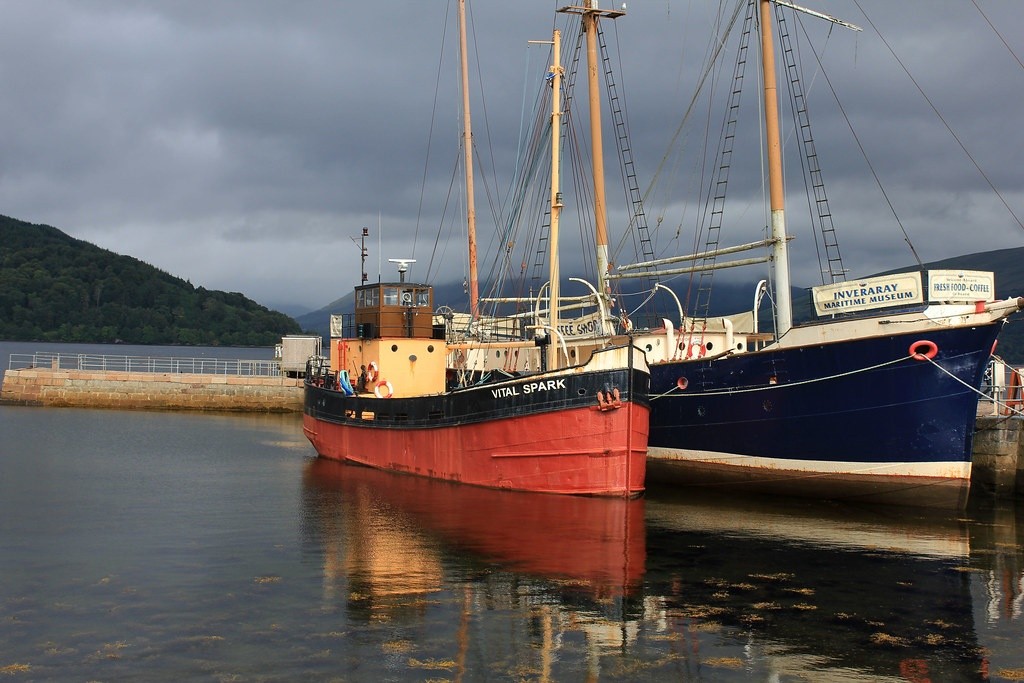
[{"left": 978, "top": 355, "right": 1001, "bottom": 404}]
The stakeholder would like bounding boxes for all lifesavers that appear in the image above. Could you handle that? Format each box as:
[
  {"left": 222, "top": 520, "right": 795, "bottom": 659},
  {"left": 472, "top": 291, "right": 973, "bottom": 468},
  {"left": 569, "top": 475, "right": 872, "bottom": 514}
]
[
  {"left": 373, "top": 380, "right": 393, "bottom": 398},
  {"left": 365, "top": 362, "right": 378, "bottom": 382},
  {"left": 686, "top": 342, "right": 706, "bottom": 358}
]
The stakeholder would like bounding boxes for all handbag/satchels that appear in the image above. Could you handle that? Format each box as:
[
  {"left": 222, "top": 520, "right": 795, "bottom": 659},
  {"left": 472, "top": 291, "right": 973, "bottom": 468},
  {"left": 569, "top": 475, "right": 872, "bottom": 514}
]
[{"left": 365, "top": 375, "right": 369, "bottom": 382}]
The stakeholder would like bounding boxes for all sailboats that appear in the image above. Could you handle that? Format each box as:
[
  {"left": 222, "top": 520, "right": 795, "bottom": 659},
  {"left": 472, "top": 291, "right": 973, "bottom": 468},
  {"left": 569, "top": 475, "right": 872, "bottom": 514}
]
[
  {"left": 609, "top": 1, "right": 1023, "bottom": 517},
  {"left": 302, "top": 1, "right": 648, "bottom": 499}
]
